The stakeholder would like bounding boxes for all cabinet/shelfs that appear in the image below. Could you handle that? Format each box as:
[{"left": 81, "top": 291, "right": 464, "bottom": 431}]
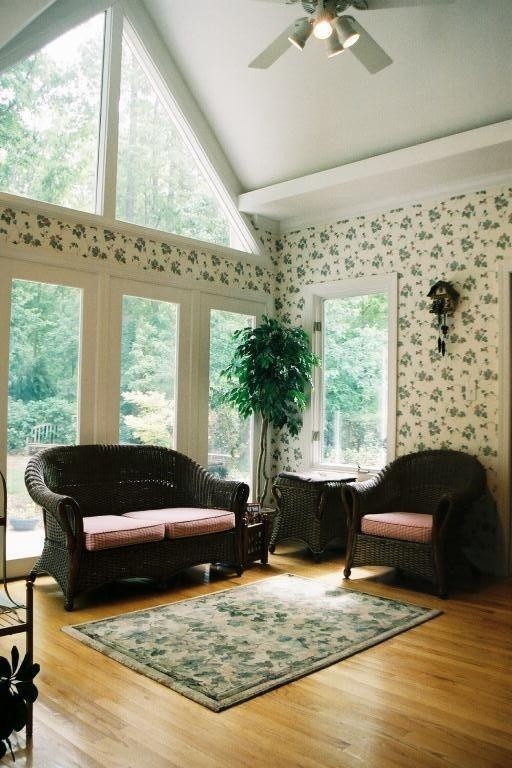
[{"left": 2, "top": 572, "right": 43, "bottom": 752}]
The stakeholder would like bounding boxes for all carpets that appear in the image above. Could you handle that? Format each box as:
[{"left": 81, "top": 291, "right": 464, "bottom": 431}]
[{"left": 60, "top": 573, "right": 443, "bottom": 712}]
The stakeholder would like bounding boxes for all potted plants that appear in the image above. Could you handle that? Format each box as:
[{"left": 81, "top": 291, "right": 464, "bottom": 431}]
[{"left": 217, "top": 312, "right": 324, "bottom": 547}]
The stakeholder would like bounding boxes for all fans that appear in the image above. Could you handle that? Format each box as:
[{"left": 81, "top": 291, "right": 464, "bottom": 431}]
[{"left": 246, "top": 0, "right": 455, "bottom": 76}]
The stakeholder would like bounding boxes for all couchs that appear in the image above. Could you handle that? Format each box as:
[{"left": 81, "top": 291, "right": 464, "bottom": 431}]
[
  {"left": 26, "top": 445, "right": 249, "bottom": 611},
  {"left": 341, "top": 448, "right": 497, "bottom": 598}
]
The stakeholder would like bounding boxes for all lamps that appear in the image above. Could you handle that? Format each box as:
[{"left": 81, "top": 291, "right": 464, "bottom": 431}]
[{"left": 288, "top": 0, "right": 359, "bottom": 59}]
